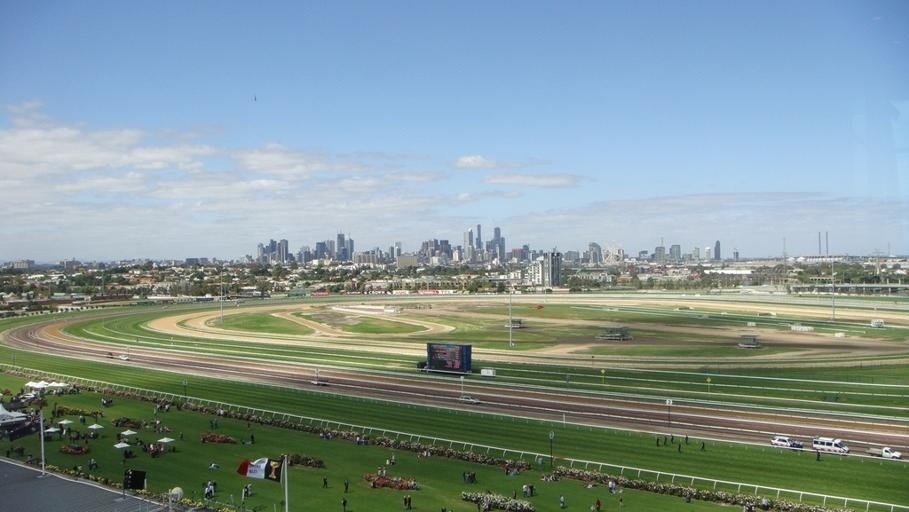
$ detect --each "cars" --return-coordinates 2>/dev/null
[458,395,480,404]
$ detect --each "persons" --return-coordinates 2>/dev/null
[1,369,858,512]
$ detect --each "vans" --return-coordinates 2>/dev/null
[812,437,850,454]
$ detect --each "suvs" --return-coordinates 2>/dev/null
[770,436,795,447]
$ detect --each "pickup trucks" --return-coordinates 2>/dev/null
[864,447,903,460]
